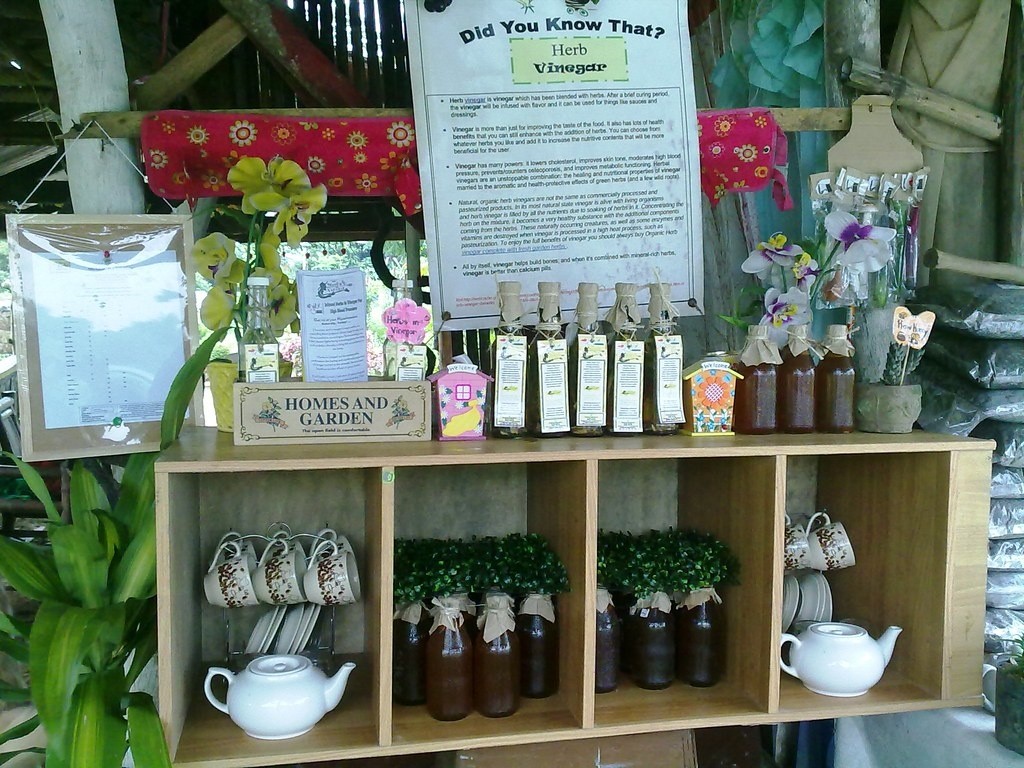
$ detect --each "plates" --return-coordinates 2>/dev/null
[245,604,322,655]
[782,572,833,634]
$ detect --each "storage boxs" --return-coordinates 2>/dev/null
[432,729,698,768]
[232,376,434,446]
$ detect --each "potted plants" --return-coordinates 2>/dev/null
[856,341,926,435]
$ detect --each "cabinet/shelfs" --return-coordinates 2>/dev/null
[154,424,997,768]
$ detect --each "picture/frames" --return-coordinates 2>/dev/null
[4,213,203,466]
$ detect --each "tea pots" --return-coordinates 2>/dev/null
[780,623,904,698]
[205,655,357,741]
[981,647,1024,716]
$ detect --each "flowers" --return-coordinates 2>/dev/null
[191,154,329,343]
[717,210,896,351]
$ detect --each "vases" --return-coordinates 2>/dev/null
[206,358,295,435]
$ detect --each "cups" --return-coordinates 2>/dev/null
[202,530,360,609]
[785,512,856,571]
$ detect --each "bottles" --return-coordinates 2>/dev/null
[394,592,557,724]
[595,587,721,695]
[491,281,683,439]
[383,280,425,381]
[733,324,856,436]
[238,277,279,383]
